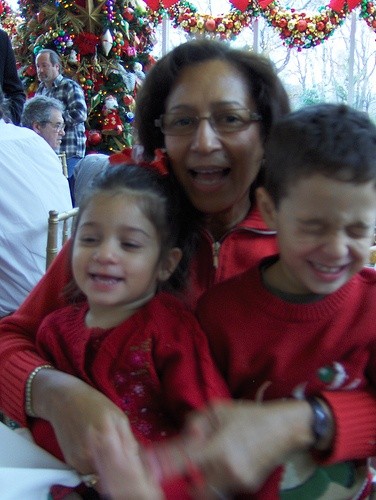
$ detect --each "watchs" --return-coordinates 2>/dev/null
[304,395,329,465]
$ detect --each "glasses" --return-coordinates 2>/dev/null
[42,122,66,132]
[155,108,261,136]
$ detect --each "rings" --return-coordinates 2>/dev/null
[83,475,101,487]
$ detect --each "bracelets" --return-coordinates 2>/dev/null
[25,364,57,418]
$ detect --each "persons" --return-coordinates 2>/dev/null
[0,38,376,500]
[0,86,73,320]
[33,49,87,178]
[19,96,67,154]
[196,103,376,500]
[0,28,27,127]
[31,160,234,500]
